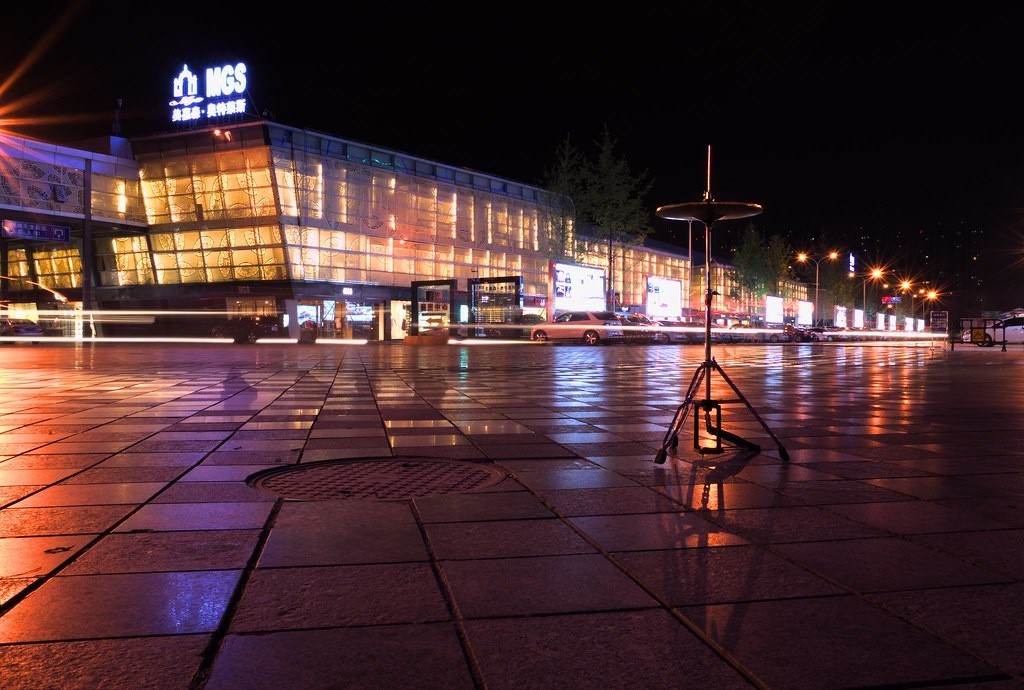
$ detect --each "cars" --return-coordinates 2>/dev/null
[805,326,947,341]
[963,317,1024,347]
[530,310,624,346]
[616,312,819,344]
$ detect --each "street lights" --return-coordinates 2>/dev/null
[847,267,884,312]
[797,249,840,326]
[885,278,940,319]
